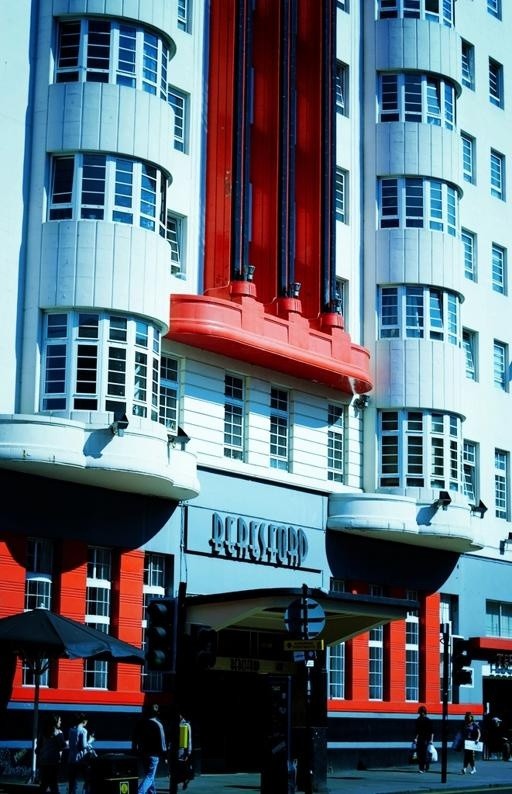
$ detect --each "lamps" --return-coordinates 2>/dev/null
[355,394,370,408]
[469,499,487,513]
[331,293,342,313]
[437,491,452,506]
[112,410,128,430]
[248,264,256,280]
[289,282,301,296]
[505,532,512,543]
[168,425,190,444]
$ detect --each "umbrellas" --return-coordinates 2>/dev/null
[0,603,146,783]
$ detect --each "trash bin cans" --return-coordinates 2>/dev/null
[91,753,140,794]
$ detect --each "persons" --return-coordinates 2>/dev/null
[488,717,511,761]
[168,709,191,790]
[132,704,167,794]
[35,714,67,794]
[70,711,94,794]
[459,712,481,774]
[413,706,436,773]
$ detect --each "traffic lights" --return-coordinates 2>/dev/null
[144,602,171,667]
[453,638,472,687]
